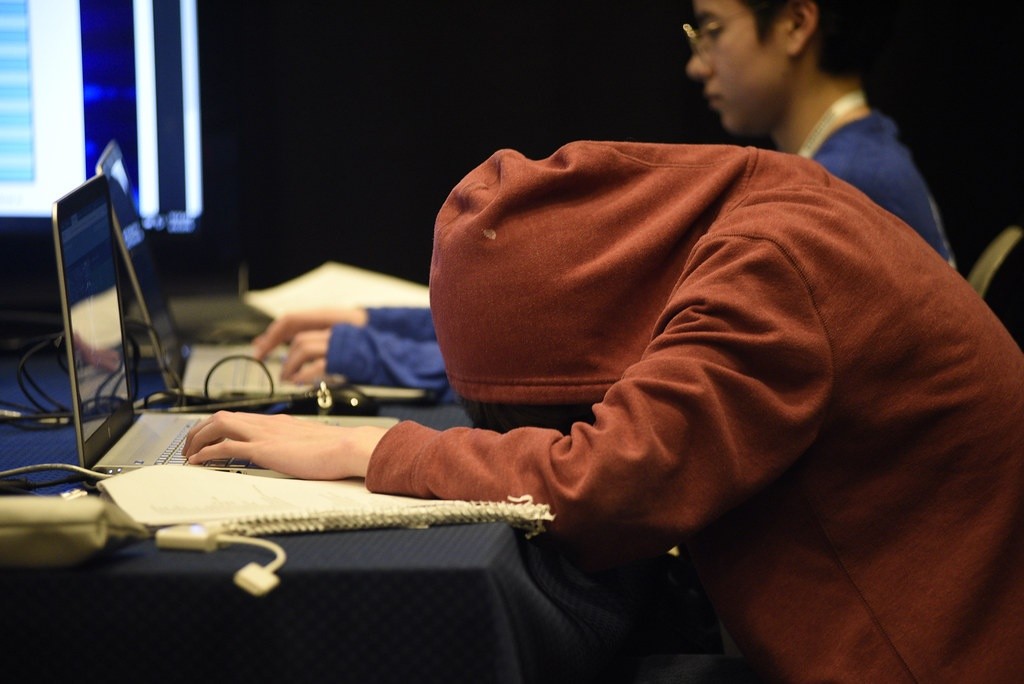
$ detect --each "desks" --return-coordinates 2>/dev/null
[0,329,594,683]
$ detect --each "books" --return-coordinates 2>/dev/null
[98,464,556,538]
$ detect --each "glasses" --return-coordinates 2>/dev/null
[683,11,752,66]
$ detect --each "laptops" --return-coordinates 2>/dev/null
[53,174,399,481]
[96,140,427,404]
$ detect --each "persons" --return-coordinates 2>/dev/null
[182,138,1024,683]
[253,0,955,400]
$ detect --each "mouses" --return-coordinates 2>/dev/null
[287,382,380,417]
